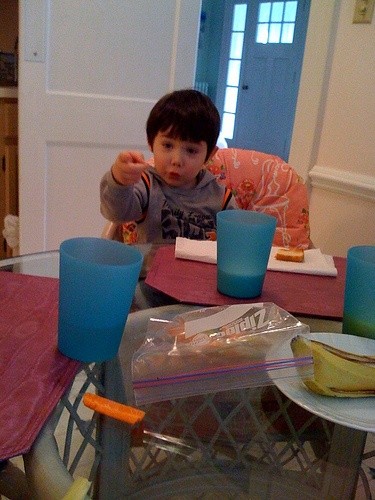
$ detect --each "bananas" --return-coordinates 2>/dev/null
[291,335,374,399]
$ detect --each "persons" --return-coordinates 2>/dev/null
[98,88,244,245]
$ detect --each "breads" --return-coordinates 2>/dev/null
[276,247,304,262]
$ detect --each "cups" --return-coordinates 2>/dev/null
[57,237,143,365]
[343,246,375,333]
[216,211,277,298]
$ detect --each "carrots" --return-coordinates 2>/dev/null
[83,393,145,426]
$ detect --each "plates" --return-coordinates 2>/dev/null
[266,332,375,433]
